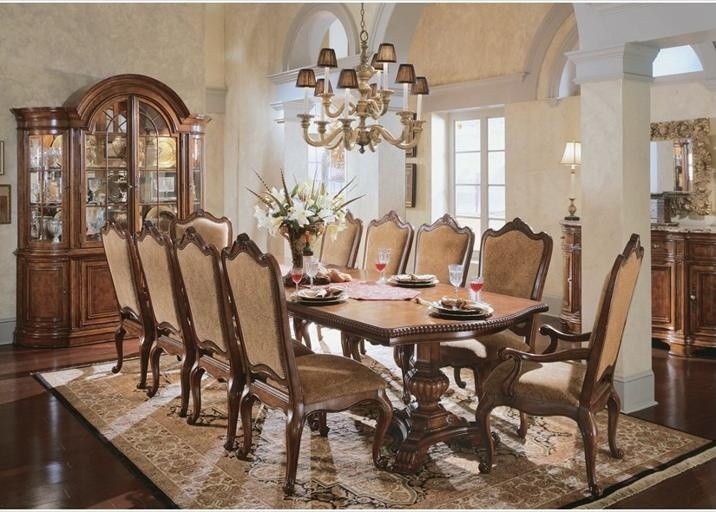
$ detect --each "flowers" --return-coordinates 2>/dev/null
[244,168,367,234]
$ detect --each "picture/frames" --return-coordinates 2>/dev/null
[0,141,4,175]
[0,184,11,225]
[403,163,417,208]
[405,112,417,158]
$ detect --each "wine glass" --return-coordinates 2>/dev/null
[374,252,388,284]
[379,249,393,283]
[470,276,484,302]
[305,258,319,288]
[88,179,99,204]
[290,268,304,291]
[448,263,464,297]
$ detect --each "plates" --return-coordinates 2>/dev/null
[291,289,348,304]
[429,300,495,319]
[388,275,438,287]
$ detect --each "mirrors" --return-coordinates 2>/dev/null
[650,135,694,196]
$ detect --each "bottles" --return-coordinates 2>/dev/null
[673,154,684,191]
[302,231,313,273]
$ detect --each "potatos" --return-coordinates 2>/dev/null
[317,266,351,282]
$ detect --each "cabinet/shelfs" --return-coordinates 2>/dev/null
[8,73,211,351]
[558,224,581,336]
[652,220,716,360]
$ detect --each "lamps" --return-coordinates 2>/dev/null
[294,3,430,155]
[560,140,581,222]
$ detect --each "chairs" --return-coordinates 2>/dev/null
[221,232,393,497]
[173,226,270,451]
[171,211,234,252]
[476,233,645,499]
[462,218,555,438]
[318,201,474,399]
[132,221,215,419]
[99,215,156,390]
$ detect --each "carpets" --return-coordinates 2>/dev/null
[31,347,716,509]
